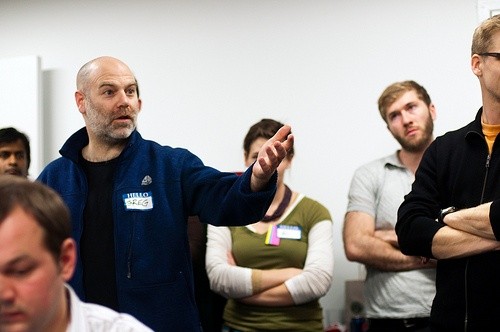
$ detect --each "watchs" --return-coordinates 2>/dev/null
[435,206,457,220]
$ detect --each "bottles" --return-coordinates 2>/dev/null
[350,315,364,332]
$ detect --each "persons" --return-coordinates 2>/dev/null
[31,56,295,332]
[342,80,441,332]
[0,127,30,178]
[1,175,156,332]
[393,15,500,332]
[206,118,333,332]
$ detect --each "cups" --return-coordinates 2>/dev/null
[326,309,344,332]
